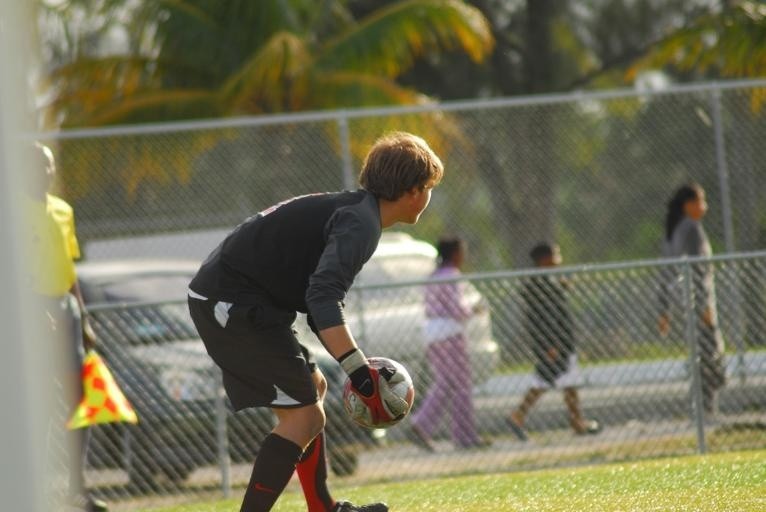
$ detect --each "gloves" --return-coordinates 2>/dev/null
[338,349,409,427]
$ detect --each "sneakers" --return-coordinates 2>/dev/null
[455,437,495,451]
[66,490,108,511]
[409,422,438,454]
[504,417,529,442]
[334,501,389,512]
[576,419,602,435]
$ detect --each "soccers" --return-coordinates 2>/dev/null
[344,357,413,430]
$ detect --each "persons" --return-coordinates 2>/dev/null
[0,139,109,512]
[186,132,445,512]
[656,184,729,425]
[504,240,605,441]
[407,236,492,453]
